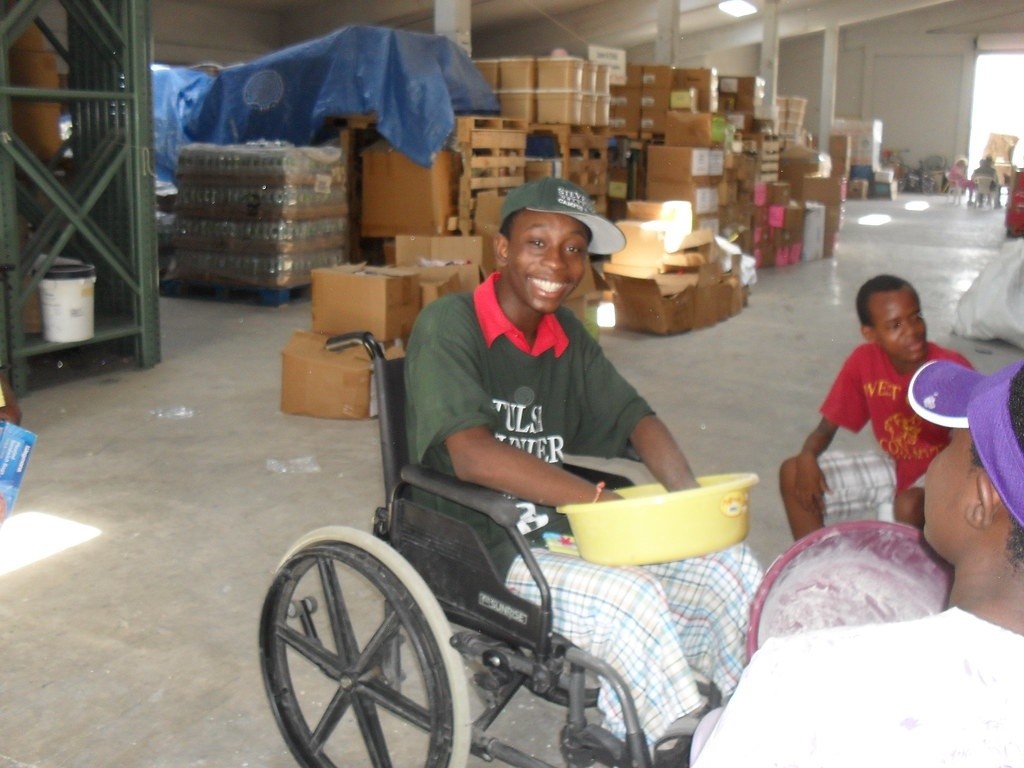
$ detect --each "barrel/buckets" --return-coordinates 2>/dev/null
[31,263,97,343]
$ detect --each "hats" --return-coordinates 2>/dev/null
[907,359,1024,529]
[499,177,626,255]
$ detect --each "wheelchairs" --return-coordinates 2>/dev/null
[248,327,719,768]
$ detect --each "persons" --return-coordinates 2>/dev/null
[685,358,1024,766]
[780,272,981,539]
[948,155,1004,209]
[400,178,760,764]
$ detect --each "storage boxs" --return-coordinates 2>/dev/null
[165,22,902,422]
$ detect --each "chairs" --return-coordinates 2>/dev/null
[972,173,1002,209]
[944,172,965,206]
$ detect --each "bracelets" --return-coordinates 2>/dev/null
[594,479,606,503]
